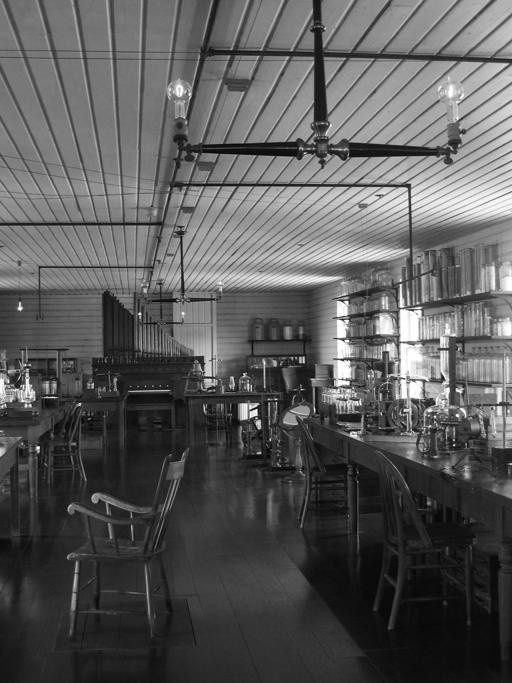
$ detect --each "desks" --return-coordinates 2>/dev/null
[62,388,130,461]
[1,399,82,537]
[184,391,285,433]
[303,414,512,683]
[0,436,23,549]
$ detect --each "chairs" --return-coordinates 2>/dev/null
[293,416,356,530]
[66,448,192,642]
[40,404,88,485]
[373,449,477,632]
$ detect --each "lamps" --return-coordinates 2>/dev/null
[137,310,186,328]
[16,297,24,312]
[140,280,224,303]
[163,76,465,165]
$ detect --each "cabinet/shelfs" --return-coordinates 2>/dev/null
[403,290,511,410]
[331,285,401,384]
[247,336,312,359]
